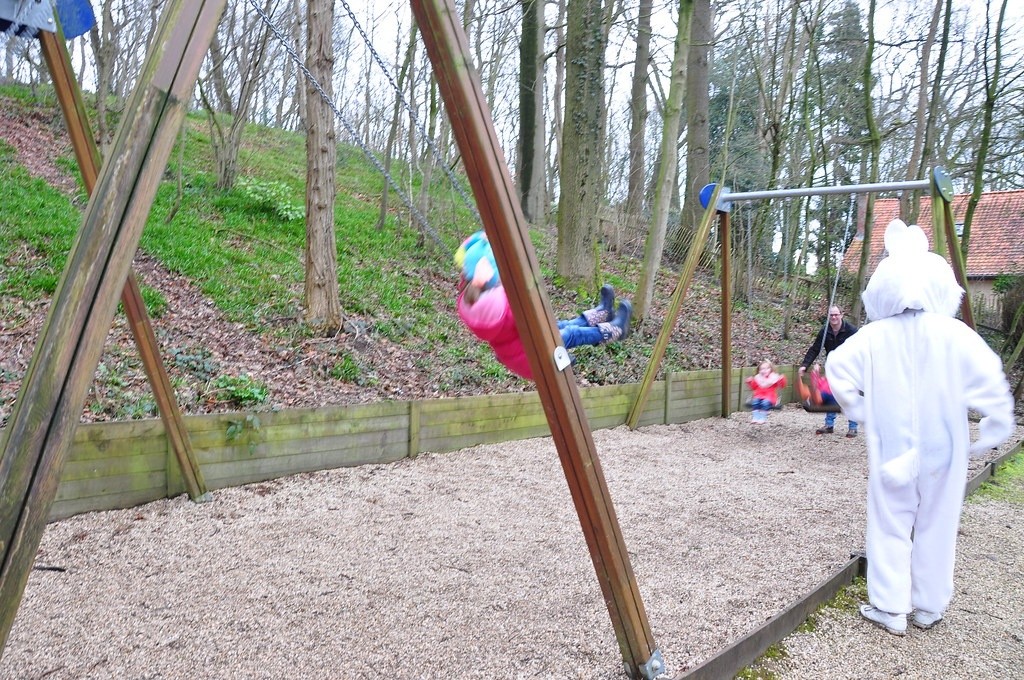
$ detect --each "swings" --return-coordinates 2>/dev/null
[744,203,792,412]
[801,198,900,415]
[250,1,577,366]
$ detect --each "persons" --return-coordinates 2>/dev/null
[798,364,837,408]
[744,359,786,424]
[450,228,633,385]
[798,305,859,437]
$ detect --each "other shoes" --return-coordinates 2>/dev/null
[846,429,858,438]
[816,425,833,434]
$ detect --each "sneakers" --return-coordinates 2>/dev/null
[913,609,942,629]
[861,605,907,635]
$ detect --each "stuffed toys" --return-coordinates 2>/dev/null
[825,218,1014,637]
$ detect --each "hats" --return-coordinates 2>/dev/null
[454,229,503,288]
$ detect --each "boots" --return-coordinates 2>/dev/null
[750,410,759,423]
[596,299,632,344]
[582,284,616,327]
[756,410,767,424]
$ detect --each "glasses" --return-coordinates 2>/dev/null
[830,313,841,318]
